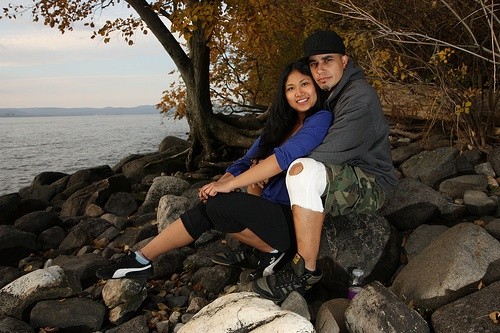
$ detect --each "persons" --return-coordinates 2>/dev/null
[212,30,398,301]
[96,61,333,281]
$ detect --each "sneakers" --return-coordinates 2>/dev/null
[212,249,254,267]
[95,250,155,279]
[259,251,286,277]
[254,251,323,303]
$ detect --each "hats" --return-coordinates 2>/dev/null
[297,29,347,61]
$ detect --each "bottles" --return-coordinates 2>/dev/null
[349,268,367,300]
